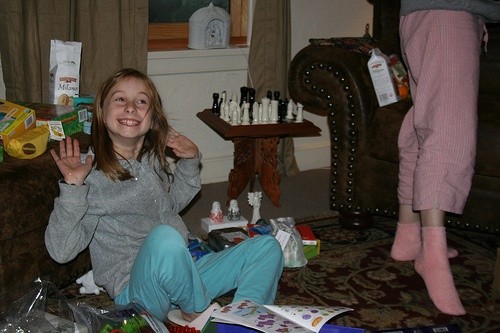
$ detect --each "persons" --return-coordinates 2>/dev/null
[390,0,500,316]
[44,68,284,322]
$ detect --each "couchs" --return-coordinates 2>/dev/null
[287,0,500,227]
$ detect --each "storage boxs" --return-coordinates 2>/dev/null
[0,97,96,151]
[200,214,321,260]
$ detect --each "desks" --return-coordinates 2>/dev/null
[0,116,95,321]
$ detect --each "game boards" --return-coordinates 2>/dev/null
[196,108,321,138]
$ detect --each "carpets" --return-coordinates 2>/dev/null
[43,209,500,333]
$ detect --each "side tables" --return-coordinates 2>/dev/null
[195,109,322,207]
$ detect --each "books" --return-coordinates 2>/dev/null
[209,300,353,333]
